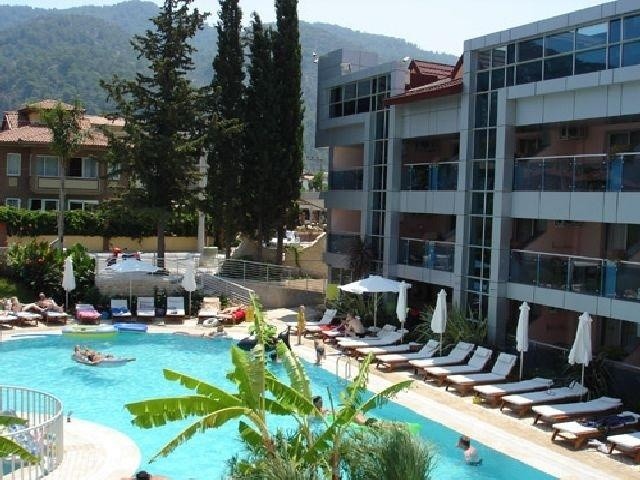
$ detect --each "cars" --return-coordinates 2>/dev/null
[268,230,301,249]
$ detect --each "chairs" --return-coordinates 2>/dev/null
[288,308,422,361]
[606,430,640,461]
[549,411,640,449]
[532,396,624,426]
[425,345,494,388]
[75,303,102,325]
[198,297,219,323]
[409,341,475,380]
[500,381,588,416]
[111,299,131,325]
[166,296,186,327]
[137,297,155,325]
[0,299,67,330]
[471,377,555,409]
[377,339,441,373]
[445,352,518,397]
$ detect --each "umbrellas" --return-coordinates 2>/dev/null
[430,289,447,357]
[395,280,408,343]
[107,255,164,308]
[337,274,411,329]
[183,255,197,313]
[61,255,76,311]
[515,301,530,380]
[568,312,593,385]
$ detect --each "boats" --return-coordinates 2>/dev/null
[76,354,136,366]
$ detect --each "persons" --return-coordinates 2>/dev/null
[75,345,103,356]
[11,296,41,313]
[345,314,365,338]
[89,354,113,361]
[38,293,63,313]
[310,395,332,420]
[297,305,307,345]
[455,434,479,464]
